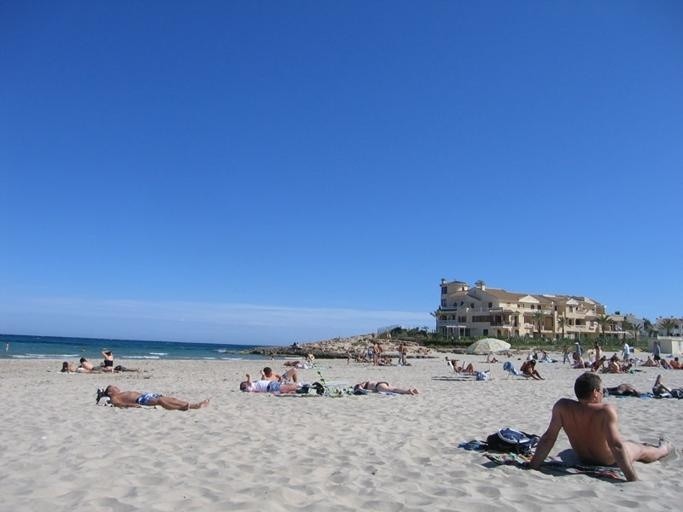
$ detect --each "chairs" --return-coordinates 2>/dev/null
[444,356,480,380]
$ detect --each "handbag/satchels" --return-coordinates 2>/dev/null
[485,425,541,455]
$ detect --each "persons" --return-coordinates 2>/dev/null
[78,358,116,373]
[62,362,101,373]
[102,350,113,367]
[528,374,668,482]
[96,385,210,411]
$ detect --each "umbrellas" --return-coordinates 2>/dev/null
[467,337,512,354]
